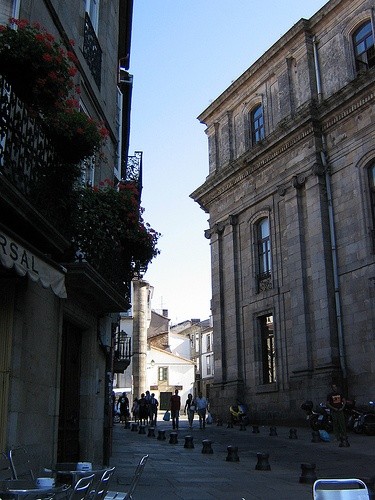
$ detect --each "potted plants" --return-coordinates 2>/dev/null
[0,15,163,306]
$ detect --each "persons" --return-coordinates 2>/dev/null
[228,402,247,423]
[133,390,158,425]
[194,391,210,428]
[165,389,181,428]
[326,383,352,448]
[183,393,198,427]
[116,392,130,423]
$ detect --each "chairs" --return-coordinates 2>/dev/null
[91,467,116,500]
[9,446,35,480]
[313,478,369,500]
[105,454,149,500]
[0,451,14,480]
[69,474,96,500]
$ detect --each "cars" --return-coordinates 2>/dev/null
[113,402,129,424]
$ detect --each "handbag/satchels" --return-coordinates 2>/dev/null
[206,413,212,424]
[319,429,332,442]
[163,410,170,421]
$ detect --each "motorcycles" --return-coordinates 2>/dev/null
[301,398,375,436]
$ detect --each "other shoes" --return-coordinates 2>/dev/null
[337,439,344,447]
[346,439,352,447]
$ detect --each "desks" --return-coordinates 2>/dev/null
[0,477,72,500]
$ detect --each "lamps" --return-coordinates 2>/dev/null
[119,329,128,344]
